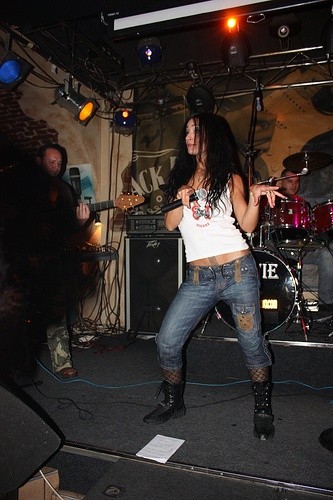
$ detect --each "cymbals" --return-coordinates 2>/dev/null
[282,150,333,175]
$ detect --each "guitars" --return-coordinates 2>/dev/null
[0,193,148,246]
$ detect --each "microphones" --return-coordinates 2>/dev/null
[161,189,208,213]
[255,78,262,111]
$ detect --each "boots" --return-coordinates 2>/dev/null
[143,380,186,425]
[252,381,275,440]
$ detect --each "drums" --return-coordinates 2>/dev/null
[311,199,333,240]
[211,247,300,337]
[264,243,271,243]
[266,200,309,243]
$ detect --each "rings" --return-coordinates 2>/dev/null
[266,191,271,193]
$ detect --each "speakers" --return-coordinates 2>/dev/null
[124,235,186,336]
[0,385,62,493]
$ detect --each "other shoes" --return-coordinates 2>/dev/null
[62,367,78,377]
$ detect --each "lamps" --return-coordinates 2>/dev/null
[0,14,250,137]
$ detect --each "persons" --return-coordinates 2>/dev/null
[259,168,333,312]
[36,144,91,380]
[142,111,286,440]
